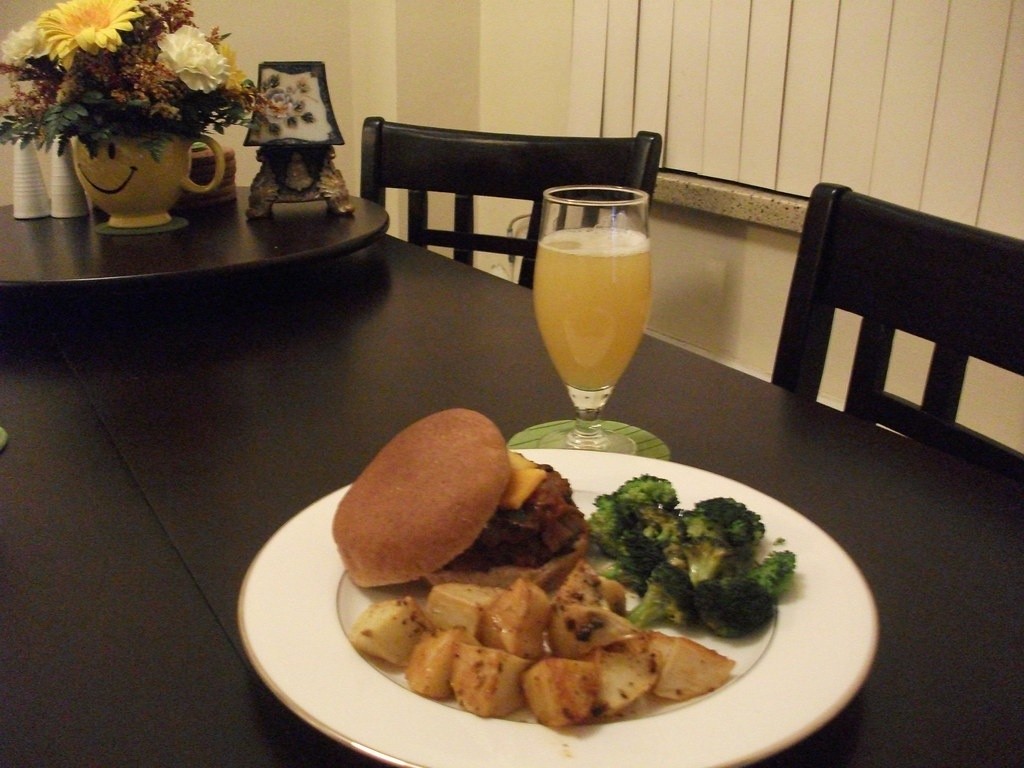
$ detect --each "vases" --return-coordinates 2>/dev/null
[68,127,227,228]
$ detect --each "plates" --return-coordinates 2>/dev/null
[238,449,879,768]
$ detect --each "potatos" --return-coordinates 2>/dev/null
[347,564,738,728]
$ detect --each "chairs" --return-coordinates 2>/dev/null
[770,182,1024,483]
[360,116,663,289]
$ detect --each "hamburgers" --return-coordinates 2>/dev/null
[331,408,588,587]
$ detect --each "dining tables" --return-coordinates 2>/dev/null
[0,231,1024,768]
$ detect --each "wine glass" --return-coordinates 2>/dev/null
[533,185,651,455]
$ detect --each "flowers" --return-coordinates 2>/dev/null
[0,0,290,161]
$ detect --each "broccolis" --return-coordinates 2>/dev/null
[590,474,796,635]
